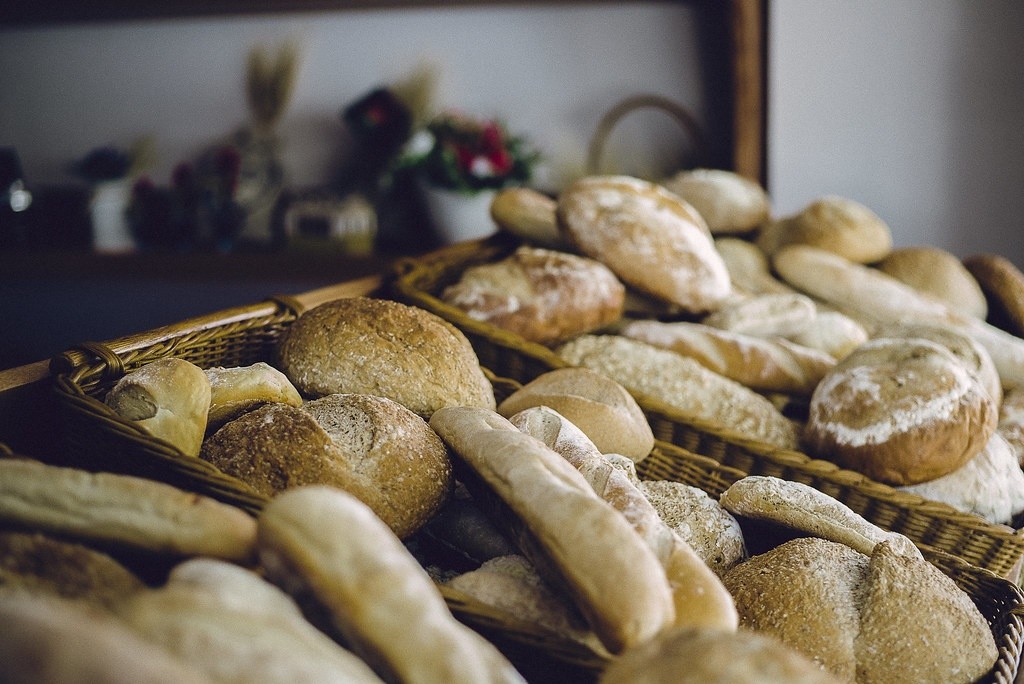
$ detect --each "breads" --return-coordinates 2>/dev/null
[0,167,1024,684]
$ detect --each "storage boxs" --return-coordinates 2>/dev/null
[1,233,1024,684]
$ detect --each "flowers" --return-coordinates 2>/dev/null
[394,110,543,195]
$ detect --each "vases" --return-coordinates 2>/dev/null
[418,172,503,249]
